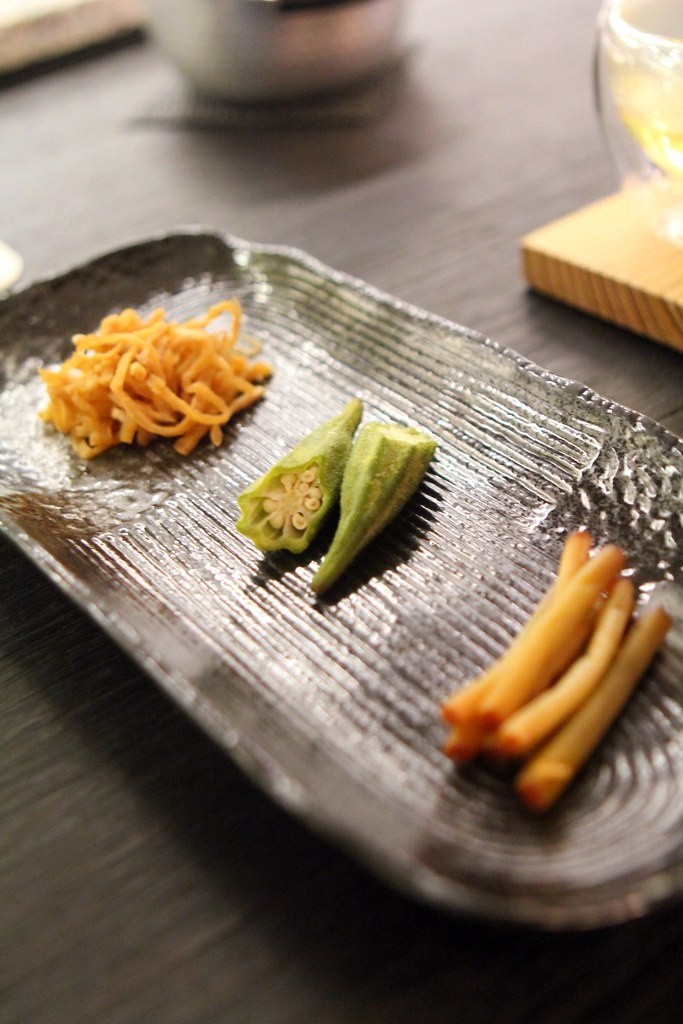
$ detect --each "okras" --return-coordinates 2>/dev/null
[310,424,437,590]
[237,400,364,550]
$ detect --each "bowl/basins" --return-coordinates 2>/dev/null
[127,0,406,104]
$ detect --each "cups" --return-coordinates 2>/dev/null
[594,0,683,247]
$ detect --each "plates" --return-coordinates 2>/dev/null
[0,227,683,928]
